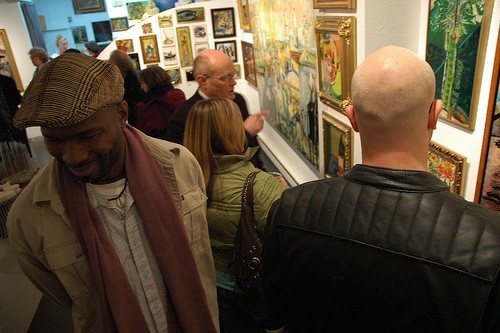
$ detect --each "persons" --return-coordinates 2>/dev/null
[6,52,220,333]
[28,35,186,145]
[266,45,500,333]
[0,75,40,178]
[173,50,267,172]
[183,98,291,333]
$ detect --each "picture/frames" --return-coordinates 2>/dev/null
[72,0,105,15]
[194,42,209,57]
[314,15,357,115]
[0,28,24,93]
[140,35,160,64]
[322,111,354,179]
[158,15,173,28]
[427,140,467,196]
[241,41,257,86]
[215,40,238,63]
[313,0,356,9]
[474,21,500,210]
[237,0,251,30]
[175,26,194,67]
[425,0,495,131]
[110,17,129,32]
[232,65,241,79]
[211,8,236,38]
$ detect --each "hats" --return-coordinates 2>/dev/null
[13,52,126,131]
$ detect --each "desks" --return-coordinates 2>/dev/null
[0,168,40,238]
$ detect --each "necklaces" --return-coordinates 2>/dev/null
[108,173,129,202]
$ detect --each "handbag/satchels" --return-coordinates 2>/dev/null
[234,171,265,296]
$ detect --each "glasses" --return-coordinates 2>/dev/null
[206,72,236,83]
